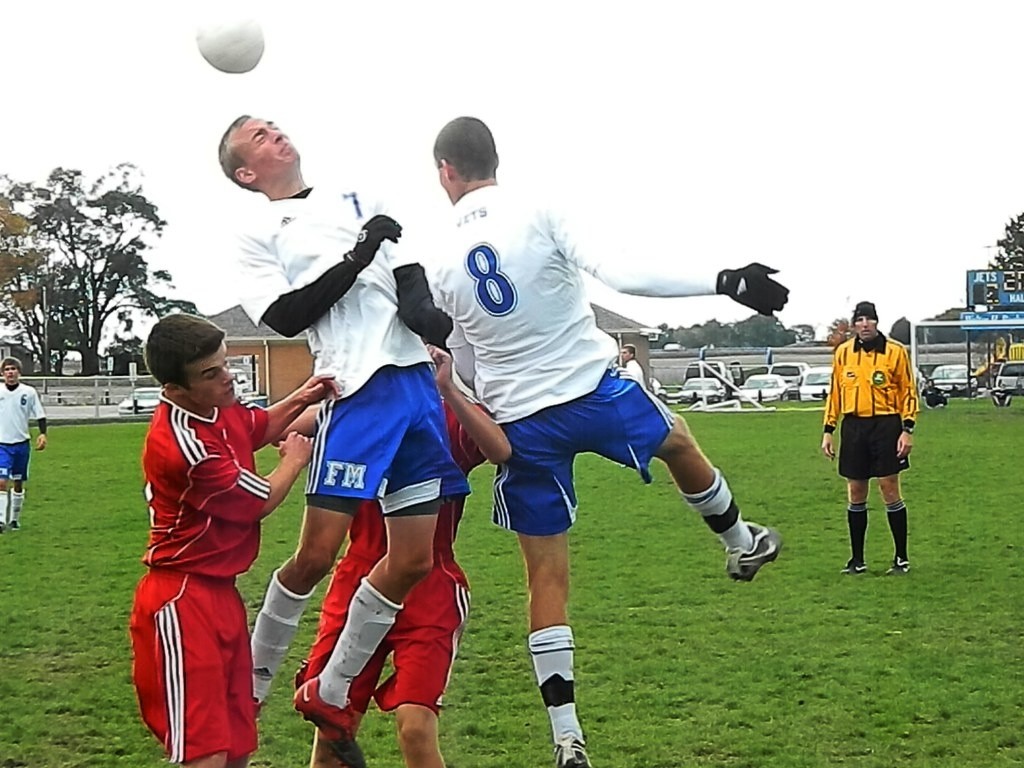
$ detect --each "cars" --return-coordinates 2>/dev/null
[798,366,836,402]
[928,364,979,400]
[647,376,668,404]
[737,373,790,404]
[994,358,1024,396]
[683,359,764,394]
[118,387,162,414]
[675,376,728,407]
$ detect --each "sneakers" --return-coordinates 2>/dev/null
[252,697,264,719]
[294,676,366,768]
[842,558,868,575]
[0,522,4,531]
[885,556,910,576]
[8,520,20,531]
[726,521,782,583]
[557,733,592,768]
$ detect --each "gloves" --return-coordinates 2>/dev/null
[344,215,402,274]
[715,262,790,315]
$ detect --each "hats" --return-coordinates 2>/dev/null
[852,302,879,327]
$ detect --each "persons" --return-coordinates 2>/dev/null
[622,344,643,386]
[0,357,47,534]
[272,343,511,768]
[425,117,790,768]
[218,114,453,768]
[820,302,921,576]
[130,313,342,768]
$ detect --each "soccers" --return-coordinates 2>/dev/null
[196,26,265,74]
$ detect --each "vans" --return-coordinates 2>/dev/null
[768,360,815,399]
